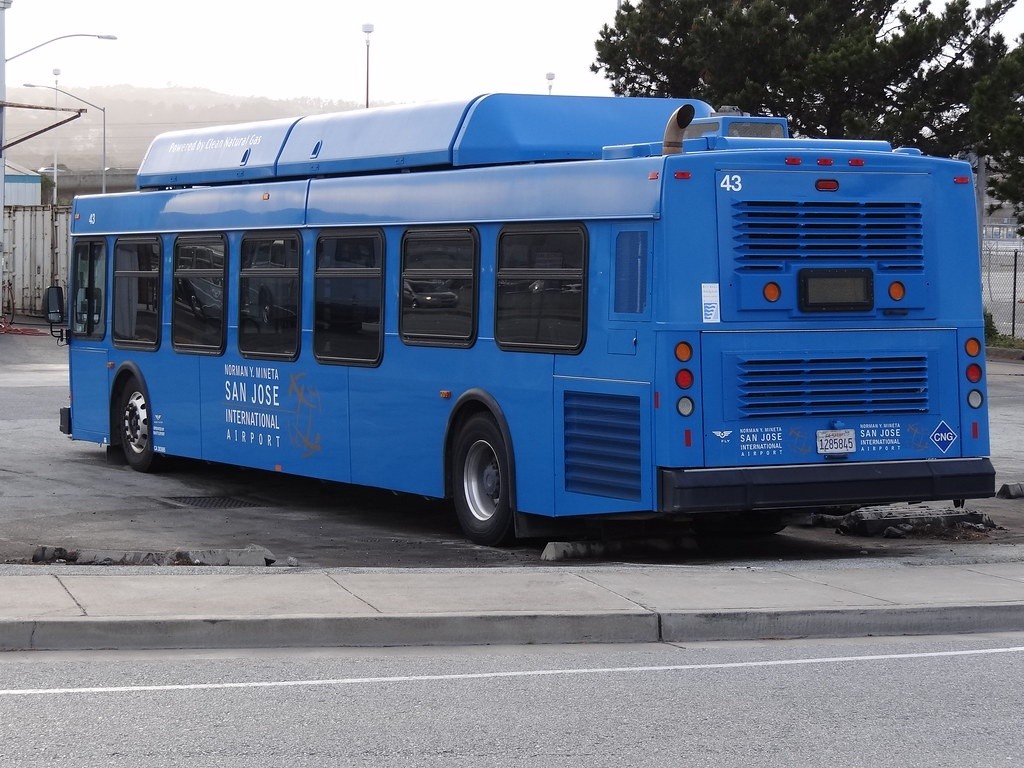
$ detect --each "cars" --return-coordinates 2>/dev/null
[497,277,583,308]
[399,276,459,310]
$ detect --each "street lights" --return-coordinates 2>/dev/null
[22,81,107,193]
[0,32,118,296]
[546,72,559,96]
[51,68,61,205]
[363,22,376,110]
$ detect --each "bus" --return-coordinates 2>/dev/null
[40,91,999,556]
